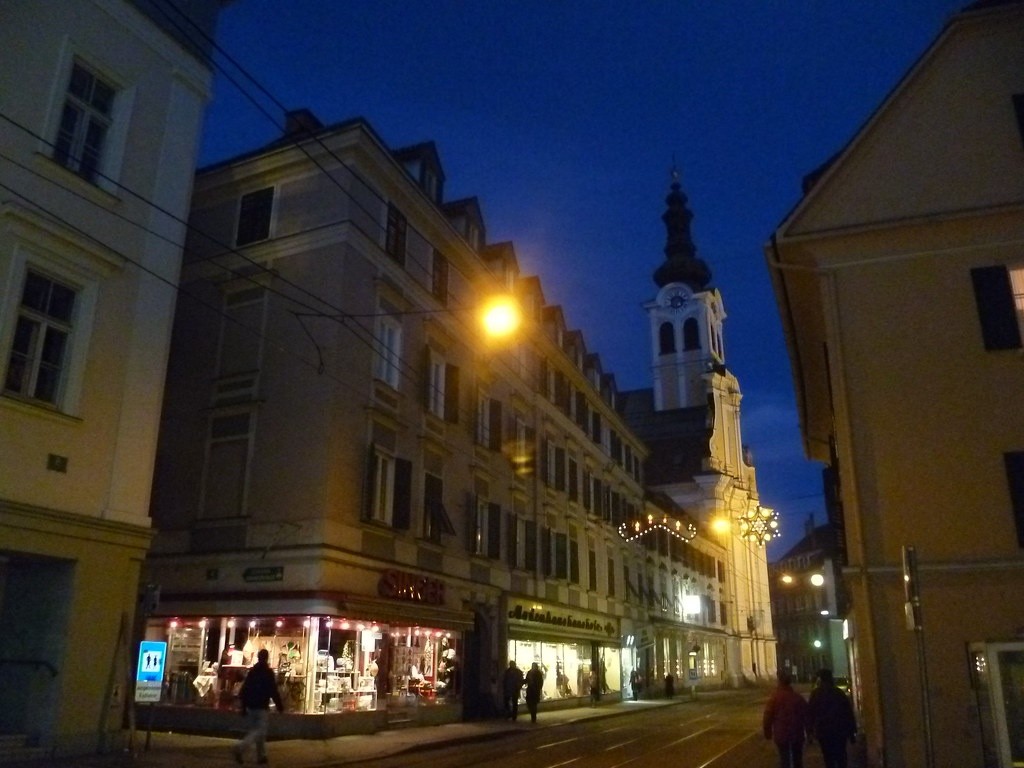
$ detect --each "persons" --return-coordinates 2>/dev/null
[523,662,543,721]
[630,670,639,701]
[665,673,674,700]
[588,671,601,707]
[502,660,523,721]
[807,667,858,768]
[763,666,812,768]
[231,648,286,766]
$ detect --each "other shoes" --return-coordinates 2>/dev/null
[258,757,267,763]
[233,748,244,765]
